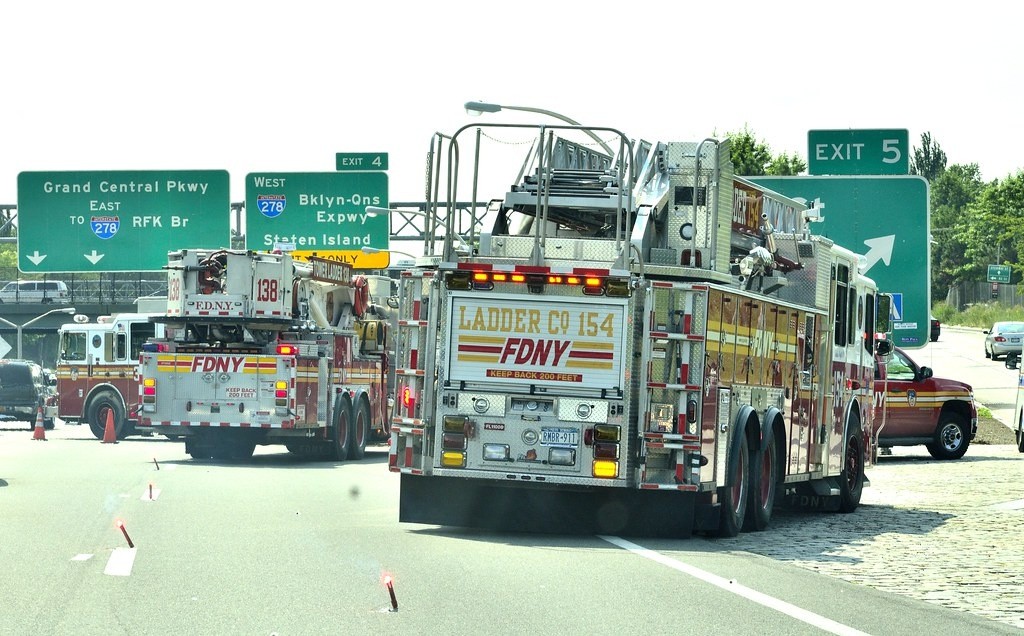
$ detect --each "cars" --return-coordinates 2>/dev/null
[929,315,941,343]
[983,321,1024,361]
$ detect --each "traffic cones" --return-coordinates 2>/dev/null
[30,406,48,441]
[100,408,119,444]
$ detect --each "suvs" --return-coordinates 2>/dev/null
[865,342,979,460]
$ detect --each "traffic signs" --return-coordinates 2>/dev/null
[986,264,1011,284]
[16,169,230,275]
[242,152,390,253]
[732,127,933,350]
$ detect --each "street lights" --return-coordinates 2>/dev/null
[0,307,76,359]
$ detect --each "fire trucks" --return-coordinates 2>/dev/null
[131,247,416,462]
[385,122,894,541]
[54,312,170,441]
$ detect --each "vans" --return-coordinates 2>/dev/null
[0,358,59,431]
[0,279,69,306]
